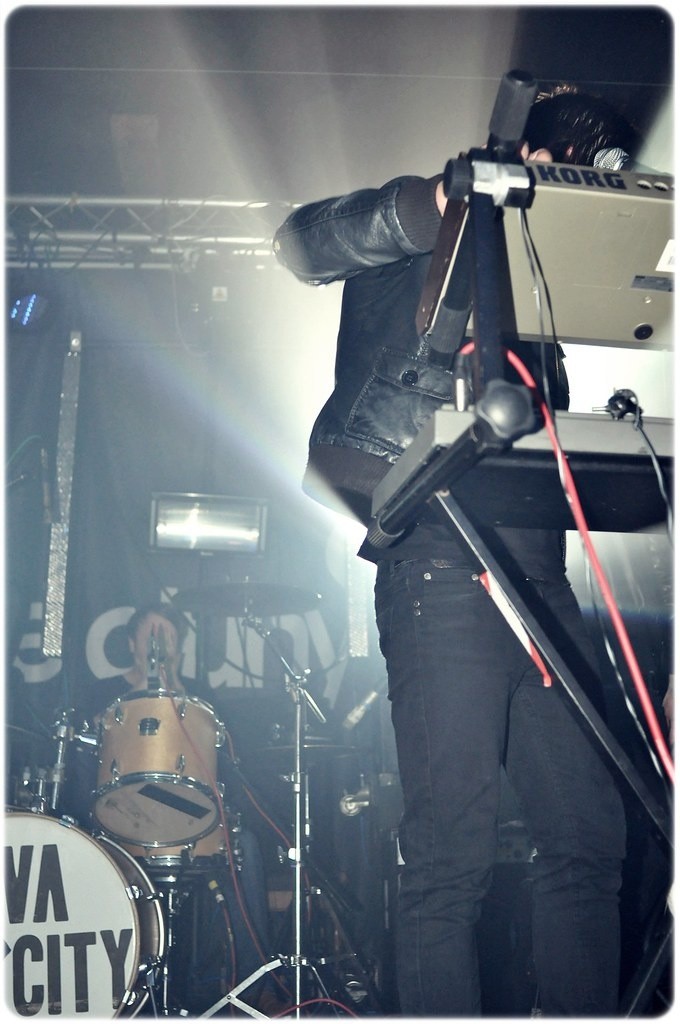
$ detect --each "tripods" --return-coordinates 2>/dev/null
[195,595,387,1020]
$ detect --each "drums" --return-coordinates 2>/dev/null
[89,688,223,849]
[119,821,234,885]
[5,812,166,1020]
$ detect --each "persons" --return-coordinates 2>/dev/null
[273,86,629,1018]
[68,604,222,839]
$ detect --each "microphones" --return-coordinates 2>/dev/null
[592,147,662,173]
[146,624,160,690]
[40,444,53,524]
[342,675,389,730]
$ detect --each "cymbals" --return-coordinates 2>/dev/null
[171,580,322,618]
[250,742,364,761]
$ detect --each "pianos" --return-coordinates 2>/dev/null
[370,398,674,536]
[414,147,676,354]
